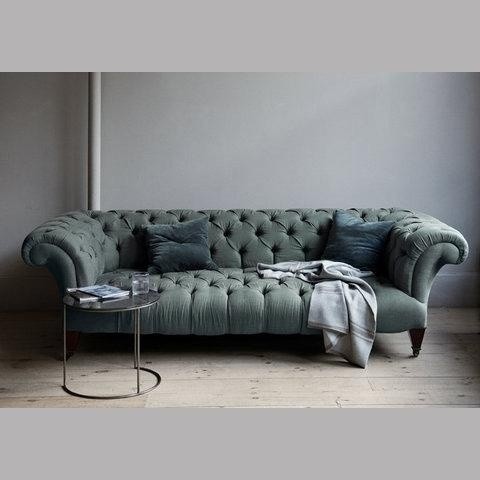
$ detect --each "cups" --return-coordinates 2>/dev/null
[132,271,149,295]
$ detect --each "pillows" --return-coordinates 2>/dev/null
[322,210,396,271]
[141,214,219,276]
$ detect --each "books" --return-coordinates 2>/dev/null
[98,297,130,303]
[66,284,130,304]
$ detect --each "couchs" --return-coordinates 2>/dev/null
[20,207,470,363]
